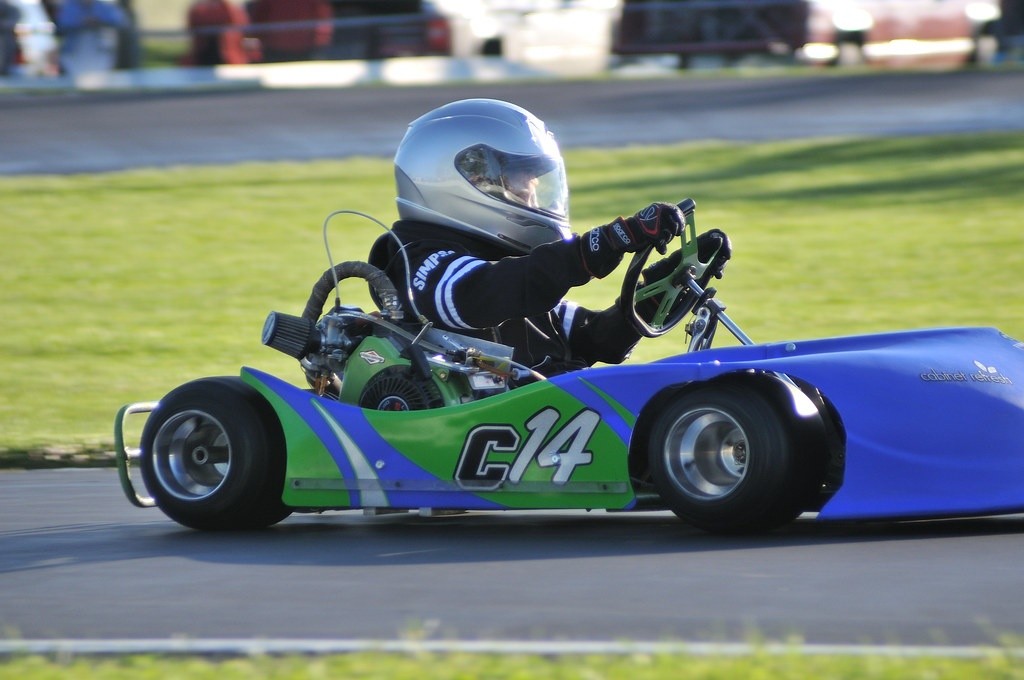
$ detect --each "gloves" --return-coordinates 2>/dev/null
[581,202,686,280]
[642,228,733,307]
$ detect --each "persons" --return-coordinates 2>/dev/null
[256,1,332,63]
[54,0,128,72]
[186,0,248,64]
[366,97,731,390]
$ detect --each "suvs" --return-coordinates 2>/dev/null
[0,0,141,77]
[609,0,1005,70]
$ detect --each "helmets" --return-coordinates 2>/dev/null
[392,97,573,254]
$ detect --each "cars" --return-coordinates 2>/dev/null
[188,0,452,61]
